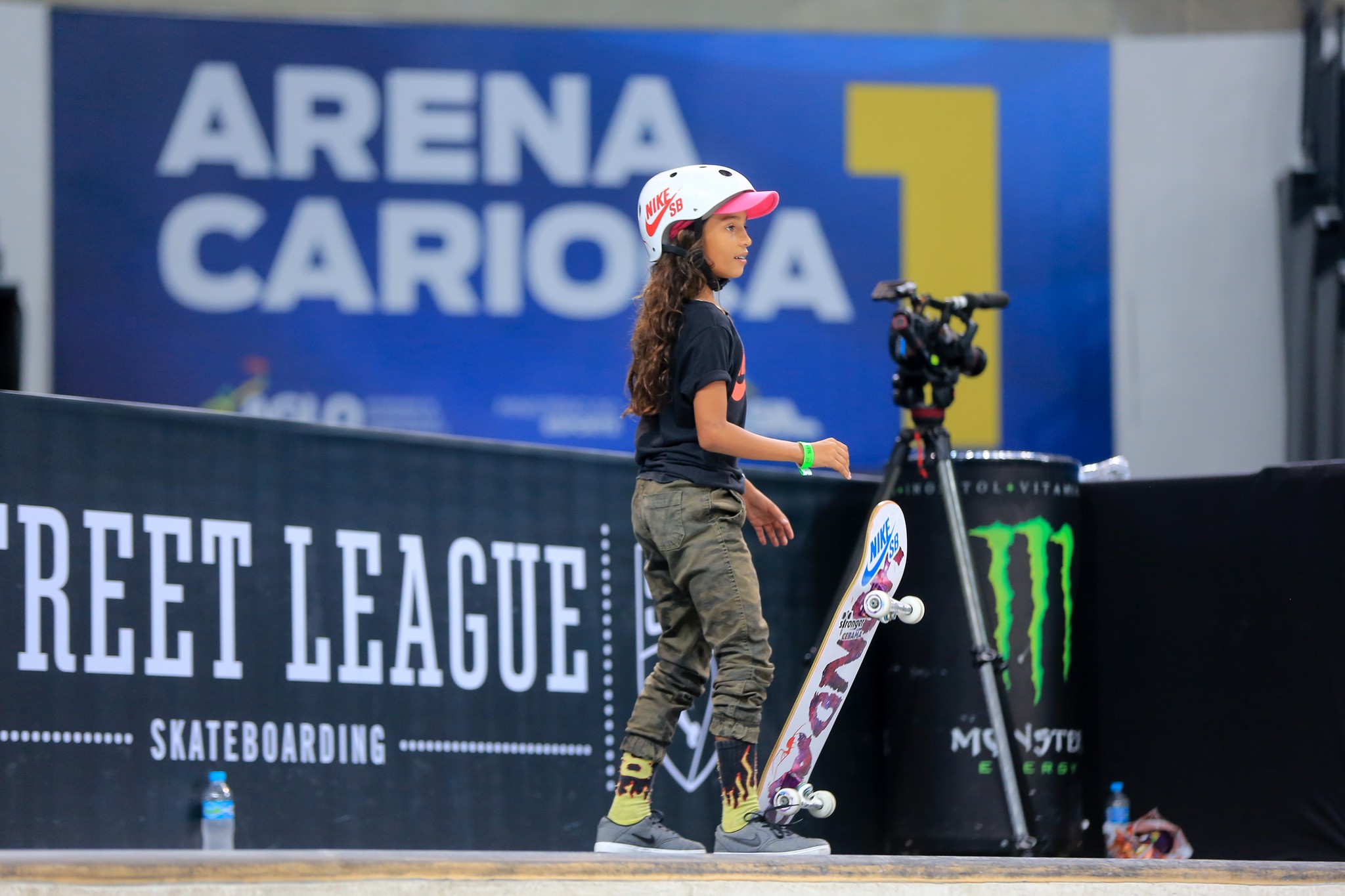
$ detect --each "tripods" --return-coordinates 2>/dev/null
[802,400,1037,854]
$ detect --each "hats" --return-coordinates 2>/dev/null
[671,189,779,238]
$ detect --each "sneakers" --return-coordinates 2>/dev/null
[713,804,831,857]
[594,809,706,856]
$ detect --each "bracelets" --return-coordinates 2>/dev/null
[795,440,814,476]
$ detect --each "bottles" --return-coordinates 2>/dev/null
[1106,782,1129,857]
[1079,455,1131,482]
[201,771,235,850]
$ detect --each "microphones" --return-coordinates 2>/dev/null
[962,291,1009,310]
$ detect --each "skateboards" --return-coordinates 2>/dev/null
[757,499,924,831]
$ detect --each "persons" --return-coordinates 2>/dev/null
[590,164,851,855]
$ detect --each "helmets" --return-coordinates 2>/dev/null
[638,165,756,262]
